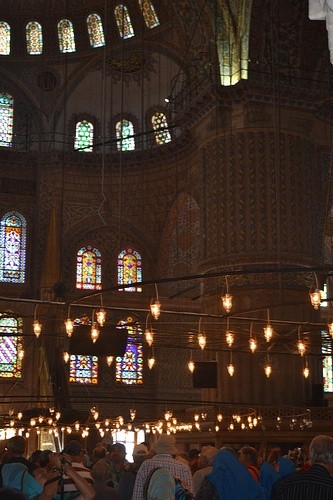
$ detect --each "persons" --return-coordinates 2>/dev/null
[0,432,310,500]
[272,435,333,500]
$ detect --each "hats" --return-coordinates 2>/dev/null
[132,444,149,456]
[153,434,178,454]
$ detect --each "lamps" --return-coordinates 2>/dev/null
[19,271,321,378]
